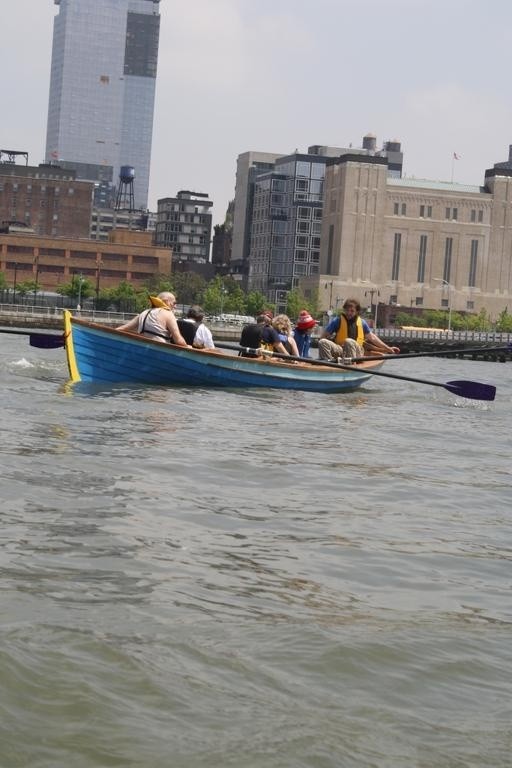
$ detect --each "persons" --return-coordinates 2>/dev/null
[292,310,316,358]
[318,298,399,365]
[240,311,299,364]
[114,291,187,346]
[170,304,214,349]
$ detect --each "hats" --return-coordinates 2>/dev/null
[297,311,315,330]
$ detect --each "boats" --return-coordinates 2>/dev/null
[62,308,386,394]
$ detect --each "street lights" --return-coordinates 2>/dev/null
[361,280,378,329]
[431,278,453,332]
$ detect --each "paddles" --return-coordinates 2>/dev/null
[30,333,67,349]
[215,343,497,400]
[308,343,512,365]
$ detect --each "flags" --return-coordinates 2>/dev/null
[453,152,461,160]
